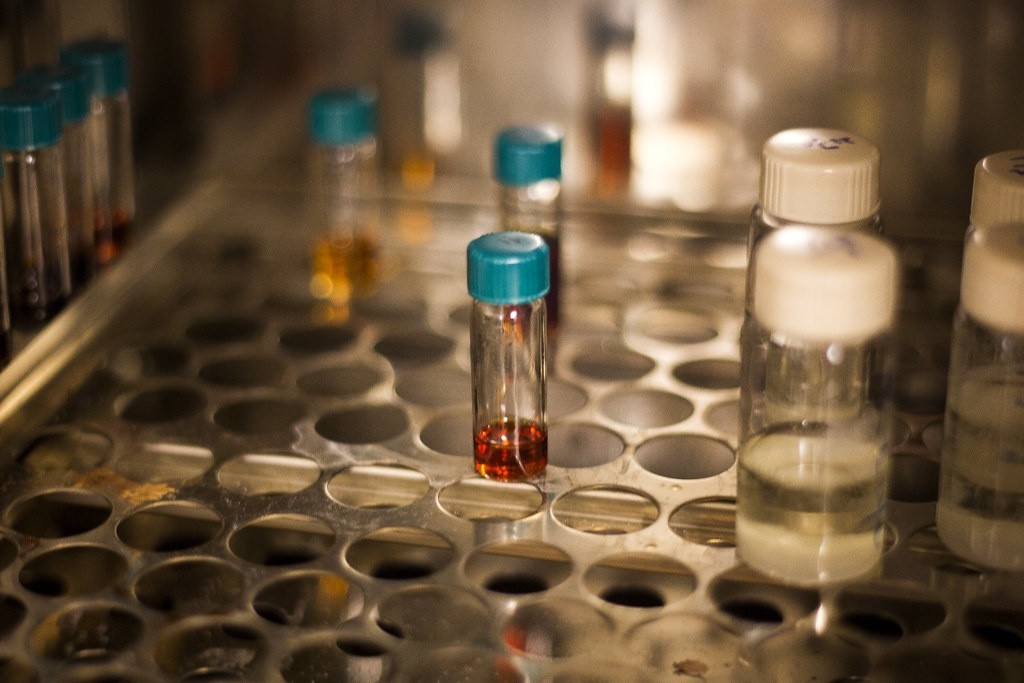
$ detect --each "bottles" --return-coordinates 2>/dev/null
[0,38,137,331]
[298,84,395,338]
[493,125,569,334]
[936,148,1024,574]
[735,127,898,588]
[466,231,551,484]
